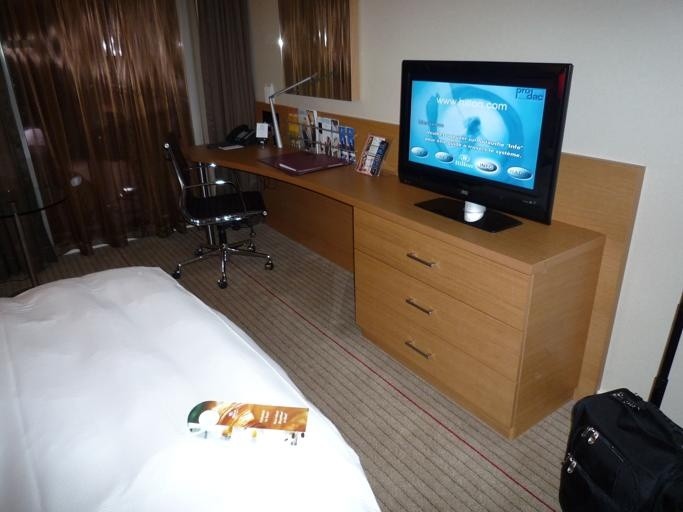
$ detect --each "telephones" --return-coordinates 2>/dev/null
[225,124,258,146]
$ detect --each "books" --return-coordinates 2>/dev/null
[272,150,346,176]
[297,106,389,177]
[177,401,310,449]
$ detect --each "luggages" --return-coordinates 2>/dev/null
[559,385,683,512]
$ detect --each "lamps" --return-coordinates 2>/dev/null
[268,71,320,151]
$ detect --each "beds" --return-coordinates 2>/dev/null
[1,264,383,512]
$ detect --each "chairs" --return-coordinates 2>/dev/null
[160,129,273,290]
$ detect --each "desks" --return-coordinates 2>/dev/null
[188,141,605,443]
[1,168,74,295]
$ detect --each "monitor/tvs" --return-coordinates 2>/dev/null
[399,60,574,233]
